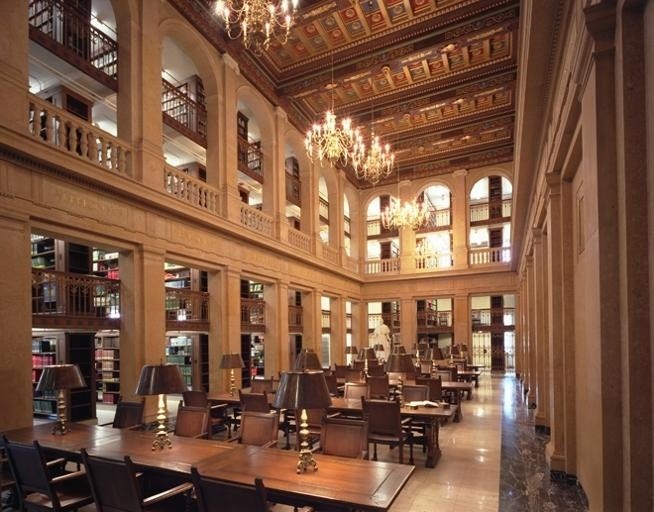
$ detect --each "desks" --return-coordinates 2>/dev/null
[203,442,415,508]
[471,361,486,382]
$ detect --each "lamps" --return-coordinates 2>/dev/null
[135,356,189,452]
[304,54,439,233]
[292,342,470,409]
[35,360,87,435]
[215,0,298,57]
[219,351,246,398]
[272,364,333,476]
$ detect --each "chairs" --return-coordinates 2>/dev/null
[0,436,274,511]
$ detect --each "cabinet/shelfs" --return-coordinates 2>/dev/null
[31,236,264,324]
[162,84,189,128]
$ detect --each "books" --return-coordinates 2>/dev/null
[31,243,59,414]
[164,262,193,386]
[91,250,120,401]
[249,279,264,381]
